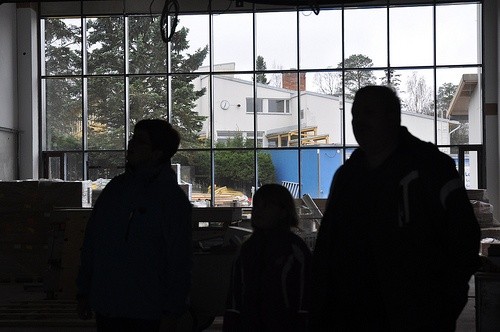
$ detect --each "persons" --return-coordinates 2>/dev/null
[75,119,193,332]
[222,86,481,332]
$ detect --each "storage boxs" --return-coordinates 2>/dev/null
[467,189,500,259]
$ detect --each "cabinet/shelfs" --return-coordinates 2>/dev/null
[189,206,253,330]
[0,179,94,298]
